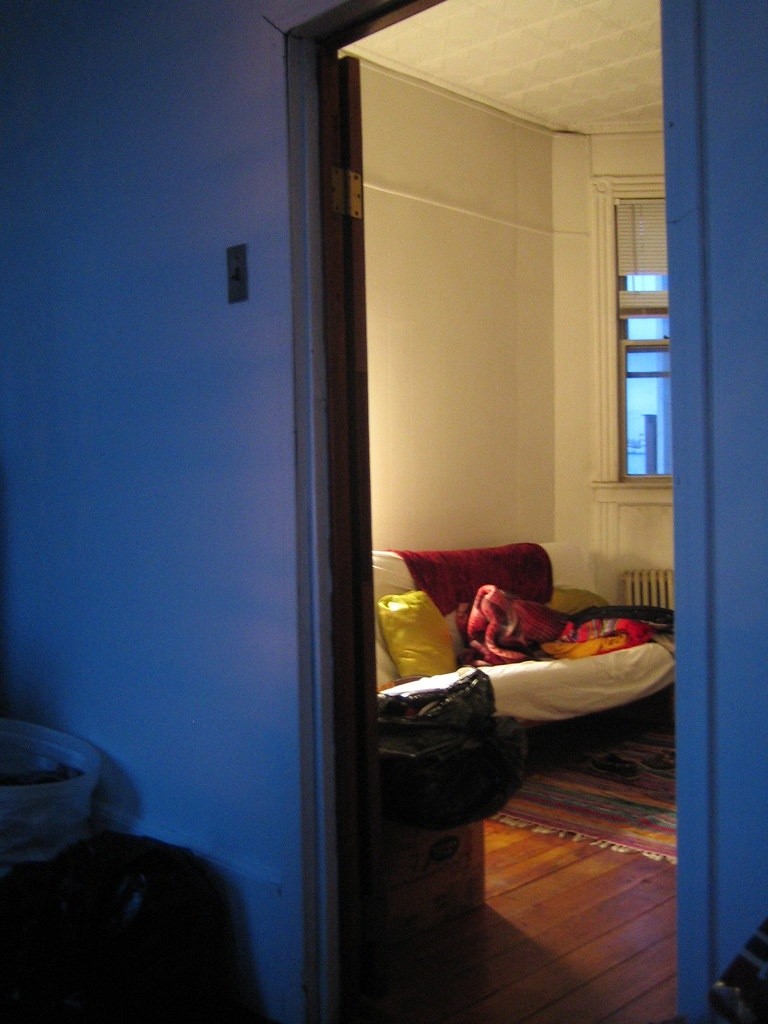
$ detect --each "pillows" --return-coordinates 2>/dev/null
[378,589,457,677]
[548,587,608,614]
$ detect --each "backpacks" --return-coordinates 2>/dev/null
[0,832,275,1023]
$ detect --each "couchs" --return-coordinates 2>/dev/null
[371,543,675,763]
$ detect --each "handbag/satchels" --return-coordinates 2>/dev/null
[378,673,498,734]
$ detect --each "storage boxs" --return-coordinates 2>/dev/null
[379,820,486,945]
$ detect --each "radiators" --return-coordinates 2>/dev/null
[619,568,674,610]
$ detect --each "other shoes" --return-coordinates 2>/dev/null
[642,749,675,768]
[580,749,638,785]
